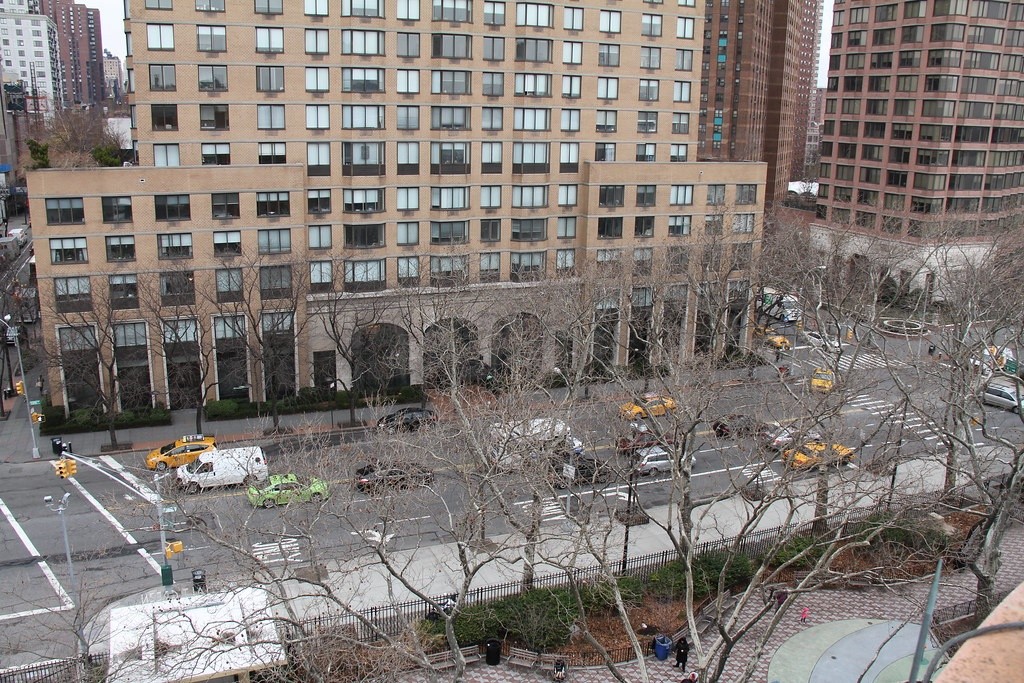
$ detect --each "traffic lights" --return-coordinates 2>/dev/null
[32,413,46,425]
[56,463,67,480]
[16,381,23,395]
[68,460,77,475]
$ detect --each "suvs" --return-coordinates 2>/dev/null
[145,437,218,471]
[542,458,612,489]
[974,379,1024,414]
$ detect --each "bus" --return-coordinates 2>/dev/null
[754,286,802,321]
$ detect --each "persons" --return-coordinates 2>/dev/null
[774,346,780,363]
[673,637,689,672]
[774,588,788,615]
[801,607,809,624]
[36,374,45,395]
[681,672,698,683]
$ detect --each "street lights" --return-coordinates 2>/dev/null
[621,422,647,577]
[796,265,826,320]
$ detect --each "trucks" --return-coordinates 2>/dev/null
[483,418,585,473]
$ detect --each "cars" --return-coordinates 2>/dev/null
[810,366,836,394]
[246,475,334,508]
[753,324,791,350]
[767,427,855,473]
[4,325,28,347]
[377,407,439,435]
[619,395,678,421]
[950,345,1014,376]
[712,413,777,441]
[352,461,435,494]
[616,429,696,477]
[801,330,842,352]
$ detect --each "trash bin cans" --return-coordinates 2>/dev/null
[653,636,672,659]
[50,436,62,453]
[192,569,207,592]
[484,638,502,666]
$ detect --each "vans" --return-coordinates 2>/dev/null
[0,229,27,260]
[170,445,268,495]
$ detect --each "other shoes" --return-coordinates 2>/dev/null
[682,668,685,672]
[673,665,679,667]
